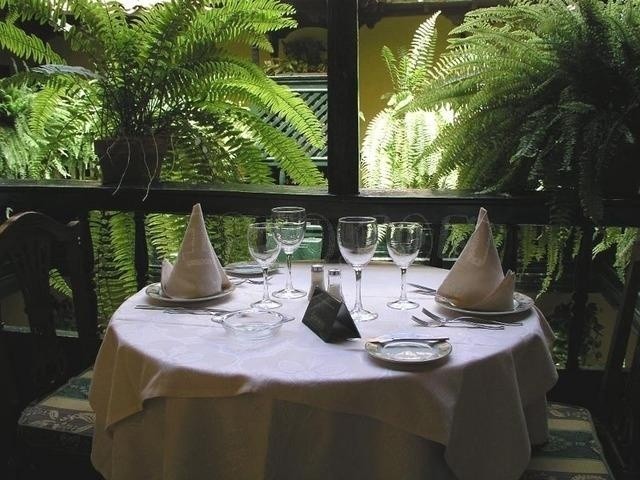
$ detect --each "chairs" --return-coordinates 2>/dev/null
[521,259,640,480]
[1,209,105,479]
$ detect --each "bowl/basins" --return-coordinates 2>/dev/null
[210,309,297,341]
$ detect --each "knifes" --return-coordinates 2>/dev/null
[346,336,451,344]
[134,304,233,315]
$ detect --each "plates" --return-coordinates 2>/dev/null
[145,281,236,304]
[225,260,279,276]
[363,334,453,368]
[434,291,536,316]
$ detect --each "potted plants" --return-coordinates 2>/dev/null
[0,0,328,188]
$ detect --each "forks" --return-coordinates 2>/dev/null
[412,307,523,331]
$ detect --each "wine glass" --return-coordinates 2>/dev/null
[337,216,379,322]
[386,221,423,311]
[271,206,308,300]
[247,221,283,311]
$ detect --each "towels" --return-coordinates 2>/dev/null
[436,208,516,313]
[160,202,232,301]
